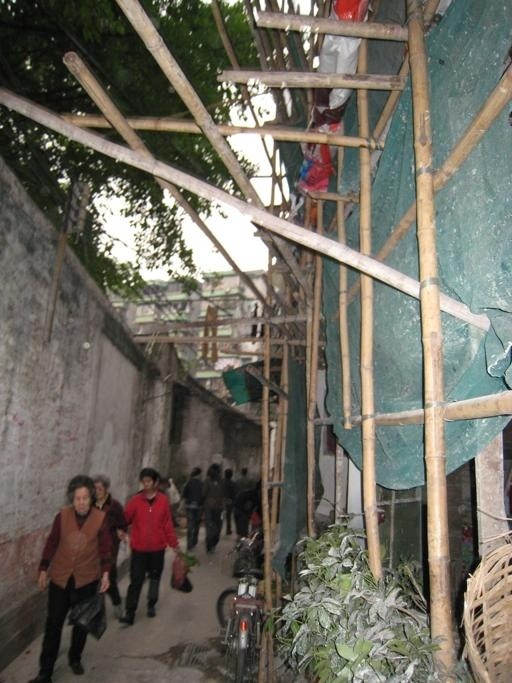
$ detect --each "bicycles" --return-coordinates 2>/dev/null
[216,527,263,683]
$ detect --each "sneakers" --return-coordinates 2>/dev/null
[110,602,156,627]
[27,672,55,683]
[67,659,84,678]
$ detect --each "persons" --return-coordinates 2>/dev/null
[87,474,127,619]
[27,474,114,682]
[157,463,274,554]
[116,467,179,626]
